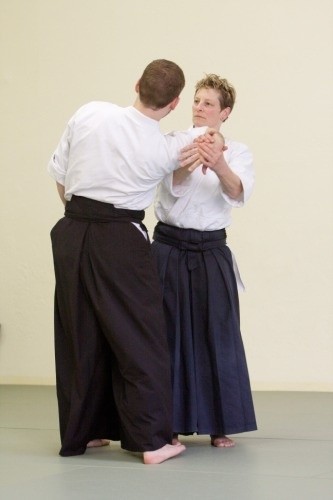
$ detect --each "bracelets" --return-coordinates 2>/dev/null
[212,130,225,148]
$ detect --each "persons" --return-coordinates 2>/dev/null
[50,57,227,464]
[142,73,258,451]
